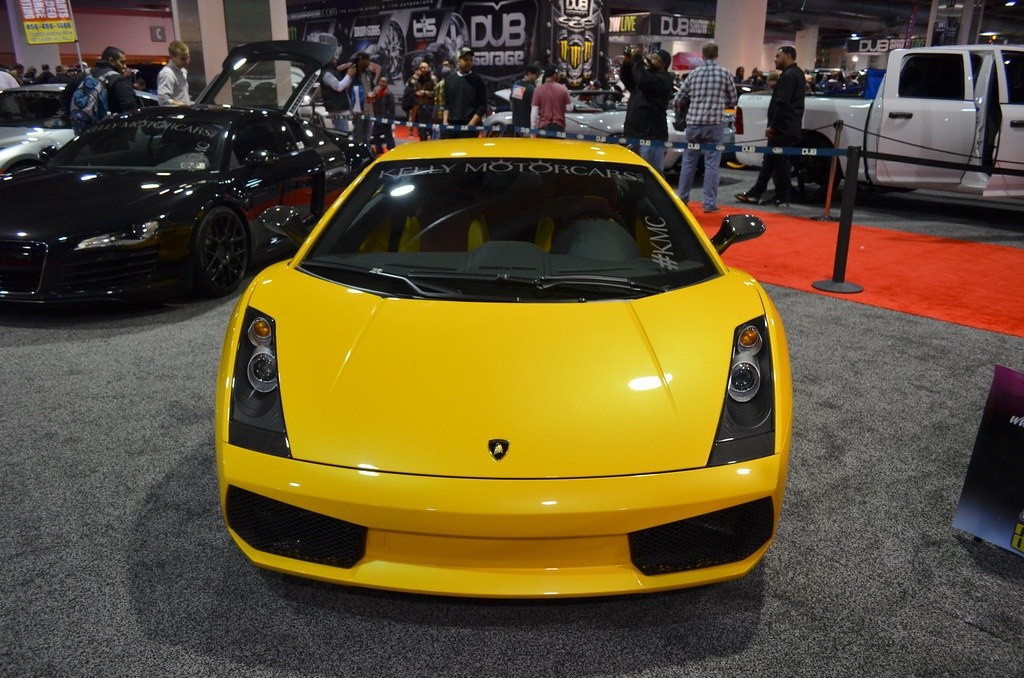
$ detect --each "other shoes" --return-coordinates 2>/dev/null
[734,192,759,204]
[760,194,791,207]
[703,207,720,212]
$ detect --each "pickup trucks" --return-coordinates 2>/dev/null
[733,30,1024,216]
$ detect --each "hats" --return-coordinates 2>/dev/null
[545,64,558,76]
[356,53,369,60]
[526,64,542,74]
[457,47,474,62]
[654,49,671,70]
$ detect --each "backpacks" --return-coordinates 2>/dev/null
[70,68,120,136]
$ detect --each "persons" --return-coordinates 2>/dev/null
[0,40,867,207]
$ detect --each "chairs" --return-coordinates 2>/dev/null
[533,179,617,255]
[398,180,491,254]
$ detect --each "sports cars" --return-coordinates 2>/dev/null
[213,133,796,605]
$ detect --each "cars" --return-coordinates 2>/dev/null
[0,38,376,322]
[481,89,727,178]
[813,67,846,91]
[1,83,165,178]
